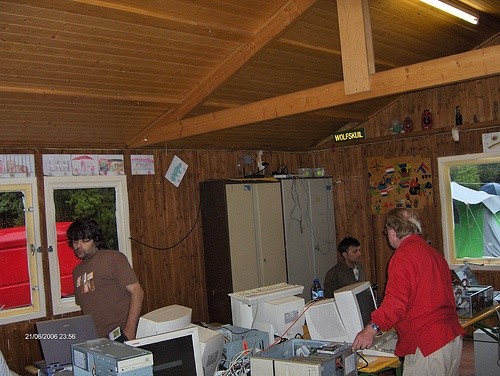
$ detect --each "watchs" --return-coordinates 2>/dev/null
[368,322,380,333]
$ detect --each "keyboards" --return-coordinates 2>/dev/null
[361,334,400,359]
[227,282,305,305]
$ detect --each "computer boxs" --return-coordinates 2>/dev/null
[448,284,494,318]
[212,323,269,368]
[246,340,358,376]
[69,337,153,376]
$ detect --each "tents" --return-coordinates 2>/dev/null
[451,182,500,259]
[481,183,500,196]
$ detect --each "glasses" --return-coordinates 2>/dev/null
[383,227,392,235]
[72,237,92,243]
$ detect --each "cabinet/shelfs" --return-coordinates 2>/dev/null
[199,175,338,327]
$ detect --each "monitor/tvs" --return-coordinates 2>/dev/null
[305,280,378,345]
[134,305,225,376]
[230,296,307,341]
[124,326,206,376]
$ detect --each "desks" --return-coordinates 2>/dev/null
[301,303,500,376]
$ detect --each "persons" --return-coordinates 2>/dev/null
[352,207,467,376]
[65,217,144,343]
[323,237,367,298]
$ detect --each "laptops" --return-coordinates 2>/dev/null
[30,314,100,374]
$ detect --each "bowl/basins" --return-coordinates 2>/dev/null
[296,168,325,177]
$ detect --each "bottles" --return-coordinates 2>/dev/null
[311,278,324,300]
[403,117,413,132]
[422,110,433,130]
[456,106,462,125]
